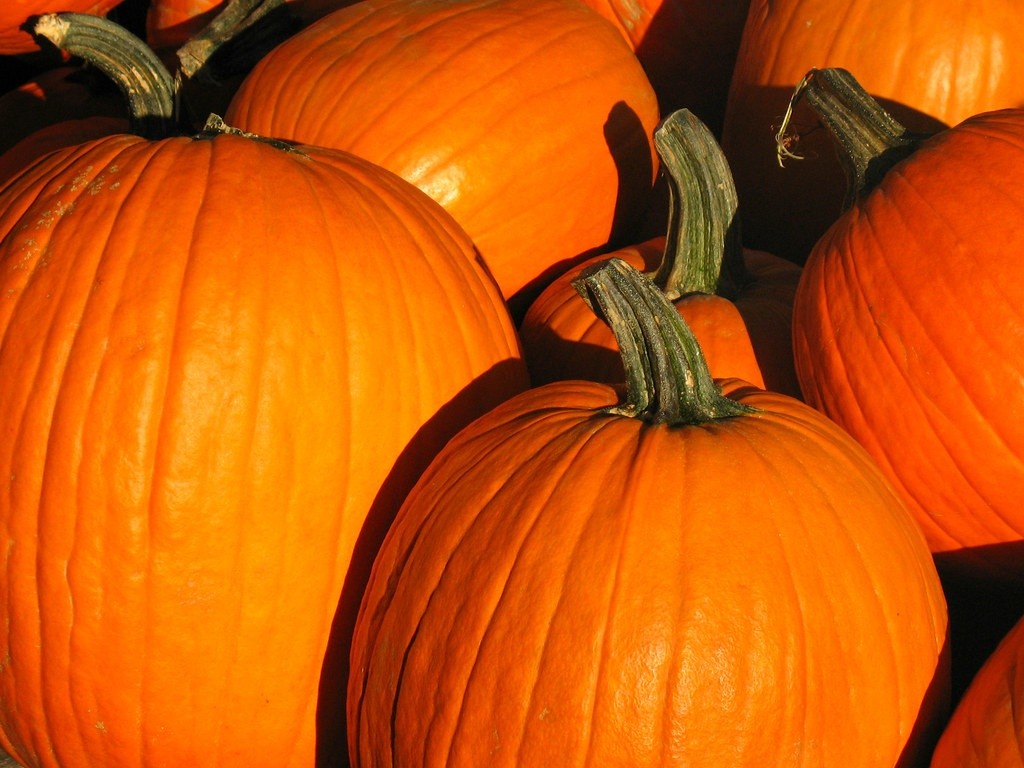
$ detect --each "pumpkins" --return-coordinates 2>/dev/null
[0,0,1024,768]
